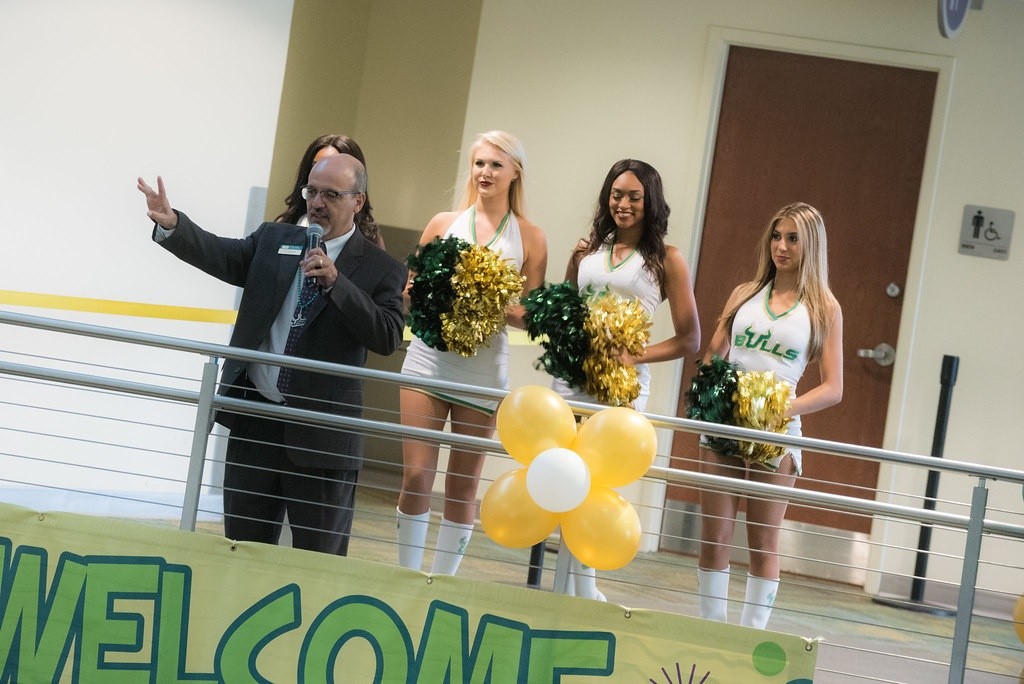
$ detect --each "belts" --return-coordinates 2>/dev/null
[245,379,288,407]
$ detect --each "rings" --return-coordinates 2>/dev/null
[320,260,324,268]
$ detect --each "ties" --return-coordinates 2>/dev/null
[276,242,327,400]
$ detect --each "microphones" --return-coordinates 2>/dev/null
[306,223,324,289]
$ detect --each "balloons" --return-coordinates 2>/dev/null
[479,386,655,571]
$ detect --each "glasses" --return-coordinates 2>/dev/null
[300,185,361,204]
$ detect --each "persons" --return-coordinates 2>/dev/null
[137,134,409,556]
[396,130,548,576]
[697,202,845,630]
[552,158,701,601]
